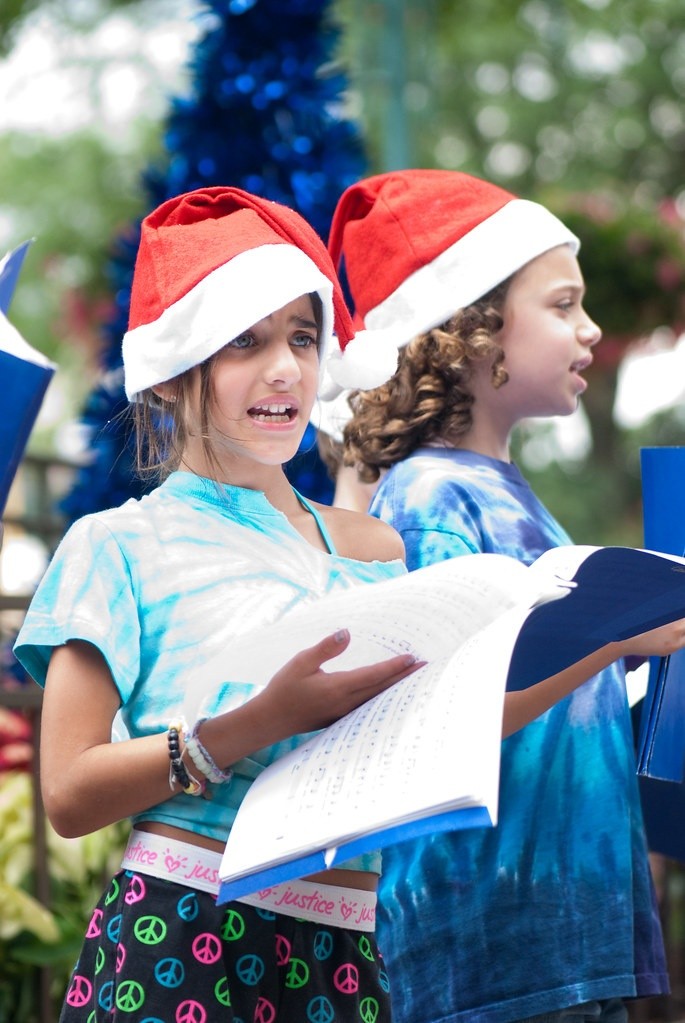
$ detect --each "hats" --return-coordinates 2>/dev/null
[123,186,400,409]
[319,169,577,403]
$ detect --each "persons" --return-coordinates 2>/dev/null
[15,184,429,1022]
[327,169,669,1023]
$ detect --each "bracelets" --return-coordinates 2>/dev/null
[168,721,205,797]
[186,719,233,783]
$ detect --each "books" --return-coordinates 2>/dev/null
[216,545,685,905]
[637,445,685,784]
